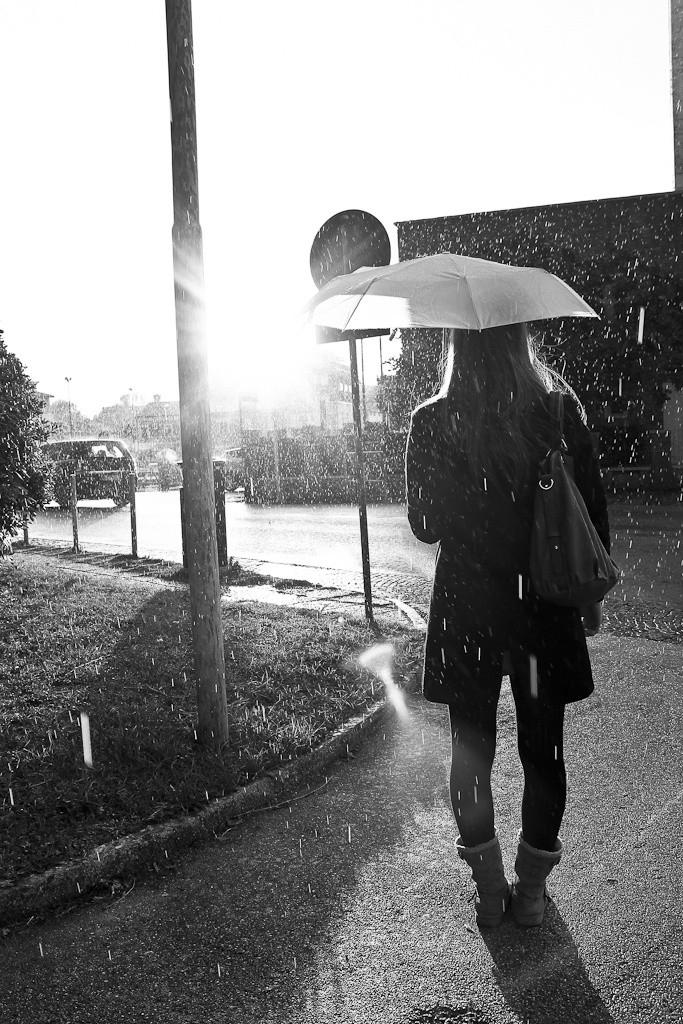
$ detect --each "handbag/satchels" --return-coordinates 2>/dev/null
[527,390,622,610]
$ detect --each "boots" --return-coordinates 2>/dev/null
[454,826,513,928]
[511,828,565,927]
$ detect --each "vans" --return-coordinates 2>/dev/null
[38,439,137,508]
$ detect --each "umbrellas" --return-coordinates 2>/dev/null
[302,251,604,339]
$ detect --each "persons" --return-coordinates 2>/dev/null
[406,317,611,929]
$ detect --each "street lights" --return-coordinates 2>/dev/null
[64,376,73,439]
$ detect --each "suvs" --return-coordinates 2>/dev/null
[216,448,245,491]
